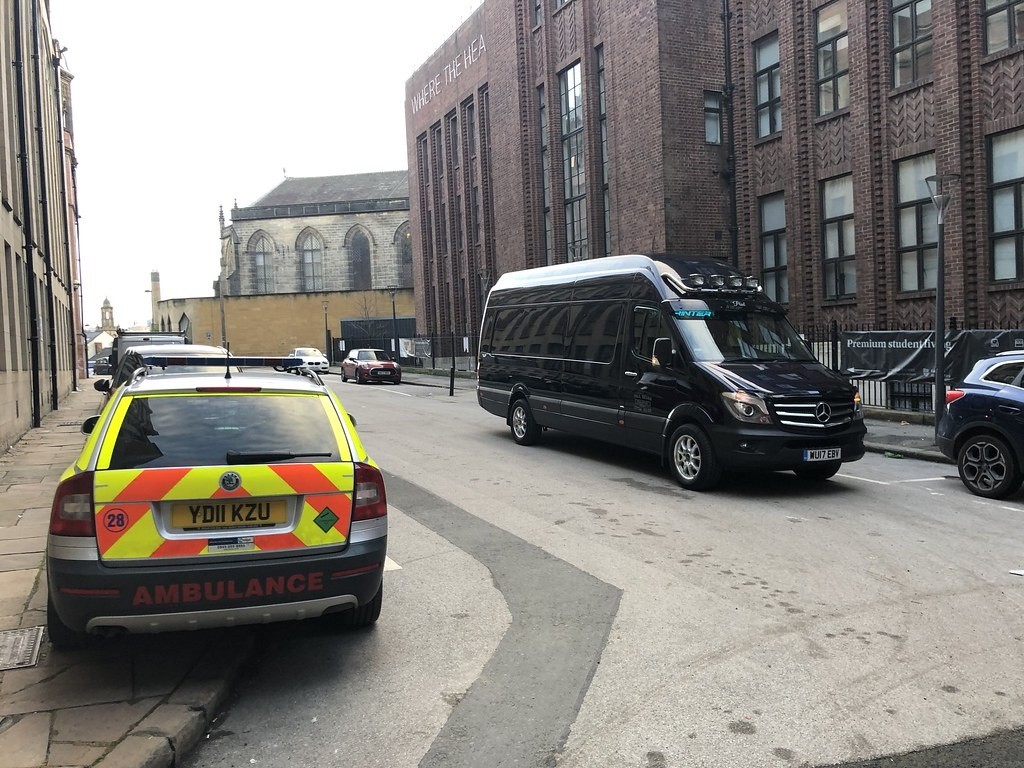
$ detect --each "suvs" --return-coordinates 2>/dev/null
[936,351,1024,500]
[93,345,244,414]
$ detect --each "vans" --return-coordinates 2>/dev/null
[474,251,869,492]
[108,328,189,378]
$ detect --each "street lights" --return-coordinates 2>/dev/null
[386,284,400,364]
[322,300,331,354]
[923,171,964,433]
[478,268,491,306]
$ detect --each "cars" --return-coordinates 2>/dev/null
[289,347,330,375]
[340,348,402,385]
[87,357,113,375]
[42,354,389,653]
